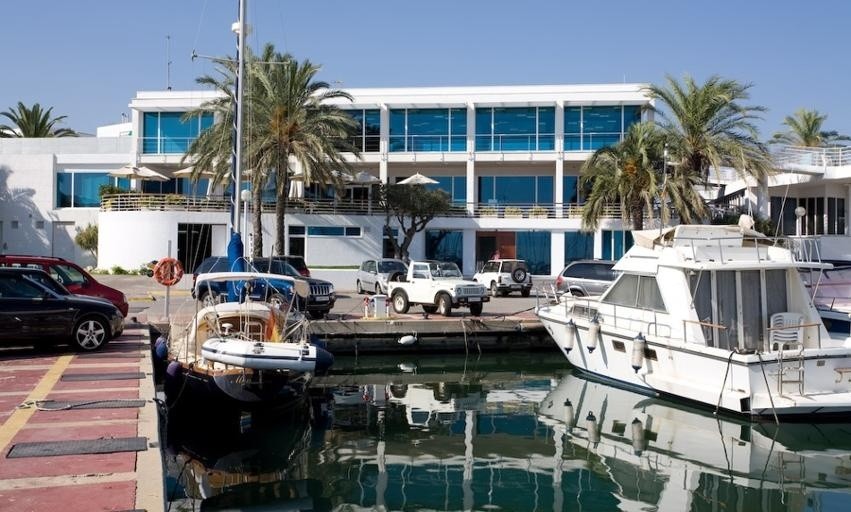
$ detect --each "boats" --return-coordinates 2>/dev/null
[161,392,330,512]
[526,373,850,500]
[532,140,851,424]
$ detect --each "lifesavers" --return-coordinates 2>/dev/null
[154,258,184,286]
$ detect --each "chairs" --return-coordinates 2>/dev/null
[768,310,804,351]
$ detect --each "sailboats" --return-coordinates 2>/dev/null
[163,0,340,417]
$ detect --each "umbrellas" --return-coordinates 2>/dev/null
[173,164,382,209]
[397,171,441,187]
[132,166,170,193]
[104,162,148,190]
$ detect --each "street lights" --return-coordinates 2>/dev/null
[238,187,255,257]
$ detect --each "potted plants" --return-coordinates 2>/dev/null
[104,194,304,214]
[479,206,549,220]
[567,204,613,219]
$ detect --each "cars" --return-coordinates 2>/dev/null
[353,256,409,297]
[268,254,312,278]
[0,253,131,357]
[555,259,622,300]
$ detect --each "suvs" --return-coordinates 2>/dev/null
[471,257,534,299]
[191,253,338,320]
[384,258,493,319]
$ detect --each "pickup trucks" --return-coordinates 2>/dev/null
[386,381,492,434]
